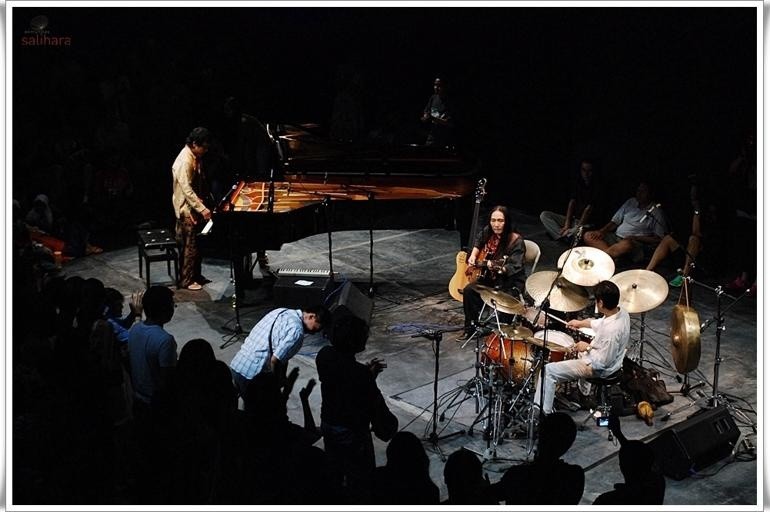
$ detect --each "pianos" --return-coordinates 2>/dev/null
[195,122,476,309]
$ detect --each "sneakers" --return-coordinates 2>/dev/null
[669,276,684,287]
[457,333,477,342]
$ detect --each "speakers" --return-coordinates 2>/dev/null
[663,405,741,473]
[326,281,377,350]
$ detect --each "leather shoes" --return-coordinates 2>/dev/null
[188,282,202,290]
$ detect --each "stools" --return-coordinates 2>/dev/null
[583,375,619,425]
[137,218,178,289]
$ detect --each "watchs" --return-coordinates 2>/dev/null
[586,344,592,354]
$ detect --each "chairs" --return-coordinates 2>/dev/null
[513,240,541,325]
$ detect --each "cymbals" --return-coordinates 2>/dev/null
[558,246,615,286]
[494,325,533,342]
[609,270,669,313]
[526,271,589,313]
[480,289,525,315]
[528,339,569,354]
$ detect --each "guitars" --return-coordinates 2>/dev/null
[465,248,508,284]
[448,178,487,302]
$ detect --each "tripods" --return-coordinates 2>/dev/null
[461,361,526,467]
[214,209,253,350]
[411,326,465,462]
[645,210,711,401]
[661,270,754,455]
[439,309,496,432]
[468,318,530,460]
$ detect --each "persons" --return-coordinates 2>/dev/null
[523,279,631,425]
[171,127,212,291]
[408,411,670,505]
[582,179,666,268]
[539,158,608,246]
[644,178,739,288]
[455,205,527,343]
[13,188,407,504]
[419,76,456,145]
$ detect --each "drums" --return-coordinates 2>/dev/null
[481,331,544,388]
[536,328,576,362]
